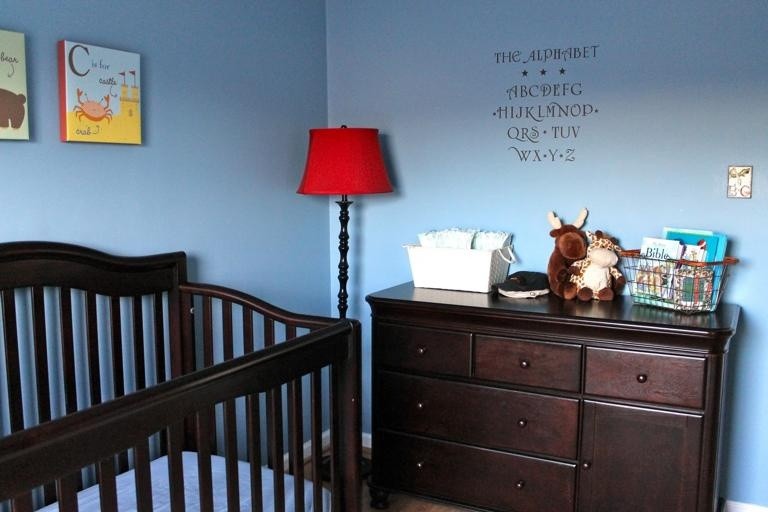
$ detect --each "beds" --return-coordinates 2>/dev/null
[0,241,363,512]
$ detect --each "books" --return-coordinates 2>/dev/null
[632,225,727,313]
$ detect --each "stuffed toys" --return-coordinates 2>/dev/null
[544,208,588,301]
[566,229,625,302]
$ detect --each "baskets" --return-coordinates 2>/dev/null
[611,244,740,313]
[402,241,518,296]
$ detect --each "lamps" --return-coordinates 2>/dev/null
[296,124,394,317]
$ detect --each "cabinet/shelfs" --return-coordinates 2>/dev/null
[364,282,742,512]
